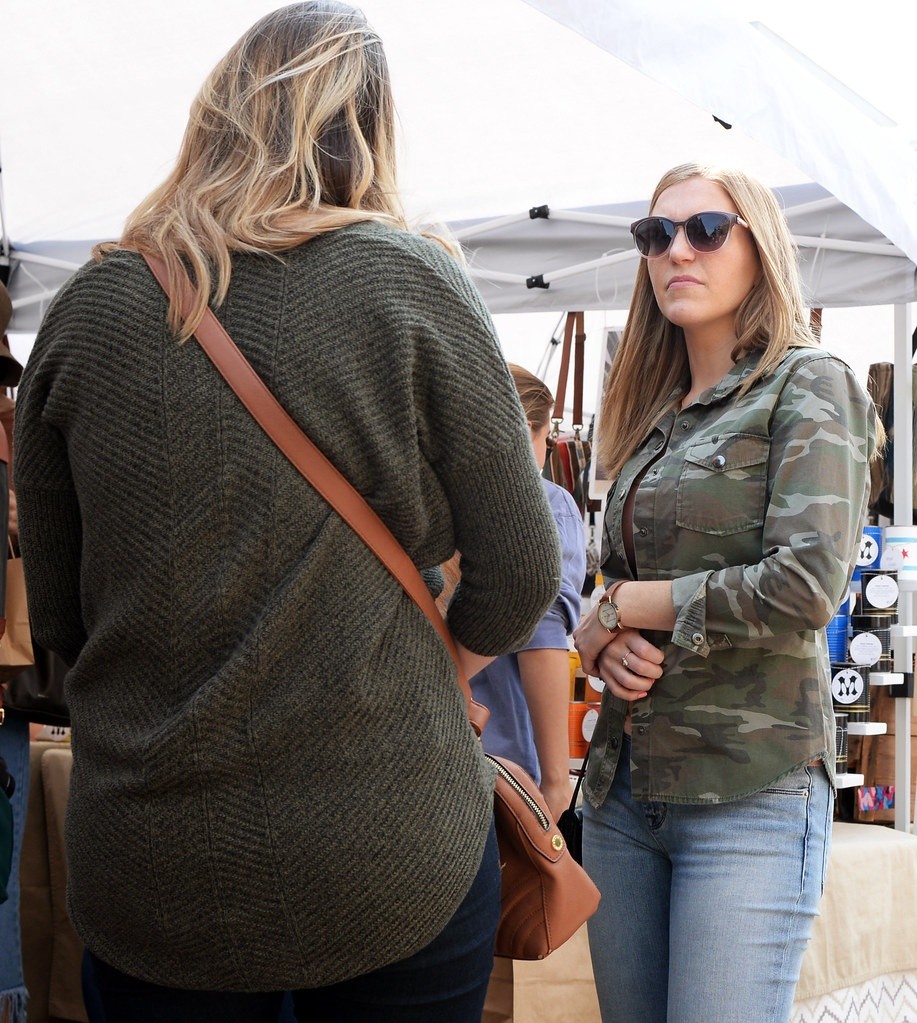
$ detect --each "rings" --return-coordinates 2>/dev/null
[621,651,634,667]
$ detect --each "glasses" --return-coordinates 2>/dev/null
[630,209,750,261]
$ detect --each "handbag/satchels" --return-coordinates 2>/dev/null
[484,754,602,966]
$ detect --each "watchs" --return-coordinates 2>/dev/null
[598,579,630,634]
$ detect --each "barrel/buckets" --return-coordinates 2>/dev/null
[825,614,850,661]
[885,524,917,581]
[851,612,892,673]
[833,583,851,623]
[831,712,850,773]
[830,661,872,722]
[852,525,882,581]
[859,567,899,626]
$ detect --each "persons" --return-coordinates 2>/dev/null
[14,1,560,1022]
[570,162,879,1023]
[467,364,587,825]
